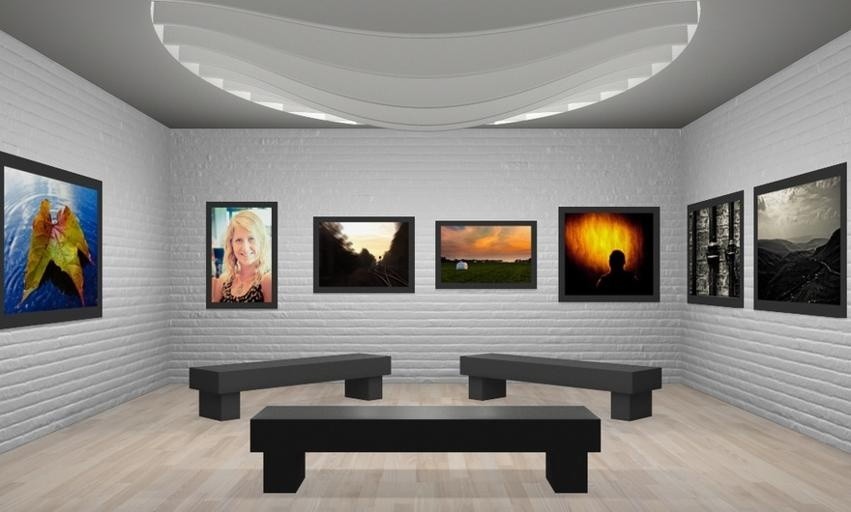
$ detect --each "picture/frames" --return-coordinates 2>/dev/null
[557,205,661,303]
[753,162,846,318]
[435,220,537,290]
[312,215,415,294]
[205,201,279,310]
[0,150,103,329]
[686,189,744,310]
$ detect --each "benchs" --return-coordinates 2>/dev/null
[188,353,392,422]
[458,352,661,422]
[246,405,601,495]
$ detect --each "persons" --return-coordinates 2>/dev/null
[594,250,641,294]
[211,210,273,304]
